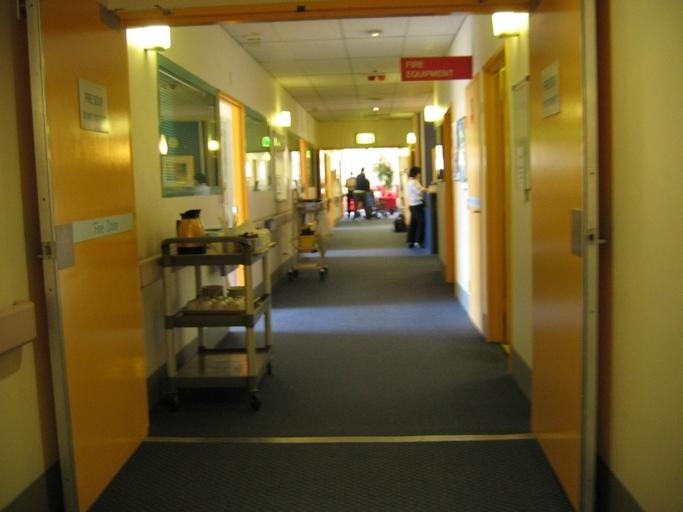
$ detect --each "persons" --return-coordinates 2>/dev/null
[405,166,432,248]
[345,167,369,219]
[192,172,211,195]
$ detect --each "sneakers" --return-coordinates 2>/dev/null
[408,241,425,249]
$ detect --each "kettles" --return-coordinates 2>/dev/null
[175,208,205,254]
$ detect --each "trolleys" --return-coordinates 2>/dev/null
[286,199,329,281]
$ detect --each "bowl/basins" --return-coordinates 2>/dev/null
[185,295,248,312]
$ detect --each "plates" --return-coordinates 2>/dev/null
[196,285,247,296]
